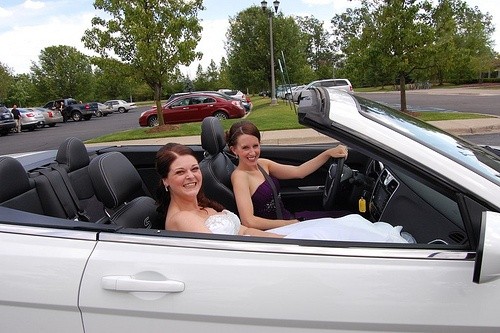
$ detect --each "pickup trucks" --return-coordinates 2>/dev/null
[43,98,98,122]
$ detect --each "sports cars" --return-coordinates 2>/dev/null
[0,85,500,333]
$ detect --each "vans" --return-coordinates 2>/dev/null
[294,78,354,105]
[166,91,250,114]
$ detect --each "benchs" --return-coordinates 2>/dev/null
[0,137,111,225]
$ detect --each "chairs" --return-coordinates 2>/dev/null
[88,151,159,230]
[198,116,239,218]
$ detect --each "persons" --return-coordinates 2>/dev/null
[156,143,418,243]
[228,121,348,229]
[79,101,82,104]
[11,104,22,132]
[58,101,67,123]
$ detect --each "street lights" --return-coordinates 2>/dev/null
[261,0,280,105]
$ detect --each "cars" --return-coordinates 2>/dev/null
[259,84,306,100]
[86,103,114,117]
[36,107,63,128]
[138,93,248,127]
[104,100,138,113]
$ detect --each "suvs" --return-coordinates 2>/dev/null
[218,89,245,102]
[0,104,46,136]
